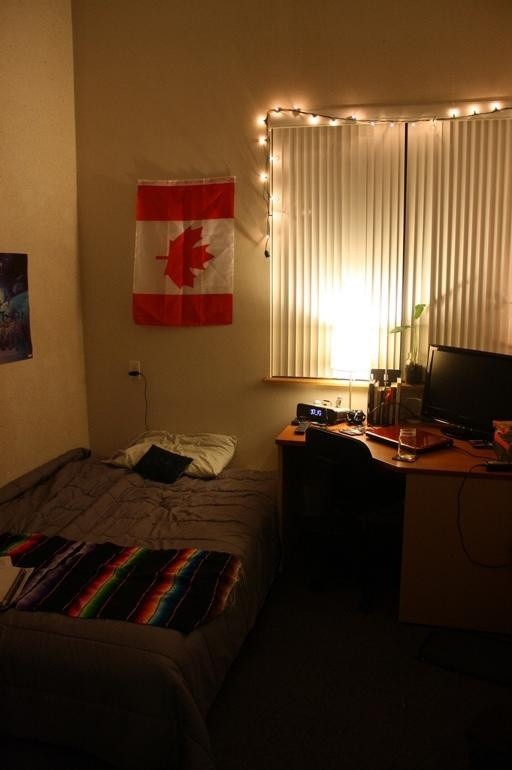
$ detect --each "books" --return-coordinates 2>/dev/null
[367,383,402,425]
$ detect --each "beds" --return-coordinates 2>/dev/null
[0,447,276,770]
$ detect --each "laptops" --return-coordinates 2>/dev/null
[365,423,453,456]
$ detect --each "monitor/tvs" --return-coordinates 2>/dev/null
[419,343,512,441]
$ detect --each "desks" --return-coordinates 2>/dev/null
[273,409,512,637]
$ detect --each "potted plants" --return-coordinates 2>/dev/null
[387,301,428,384]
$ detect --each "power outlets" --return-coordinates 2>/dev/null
[128,359,142,380]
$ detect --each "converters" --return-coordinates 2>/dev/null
[485,458,512,471]
[128,370,140,376]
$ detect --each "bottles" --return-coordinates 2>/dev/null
[398,427,417,460]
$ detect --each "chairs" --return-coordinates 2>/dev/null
[301,423,394,632]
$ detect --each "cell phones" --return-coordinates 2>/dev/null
[295,421,308,433]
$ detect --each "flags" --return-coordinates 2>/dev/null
[131,176,237,327]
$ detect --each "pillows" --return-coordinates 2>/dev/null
[102,429,241,479]
[128,443,193,484]
